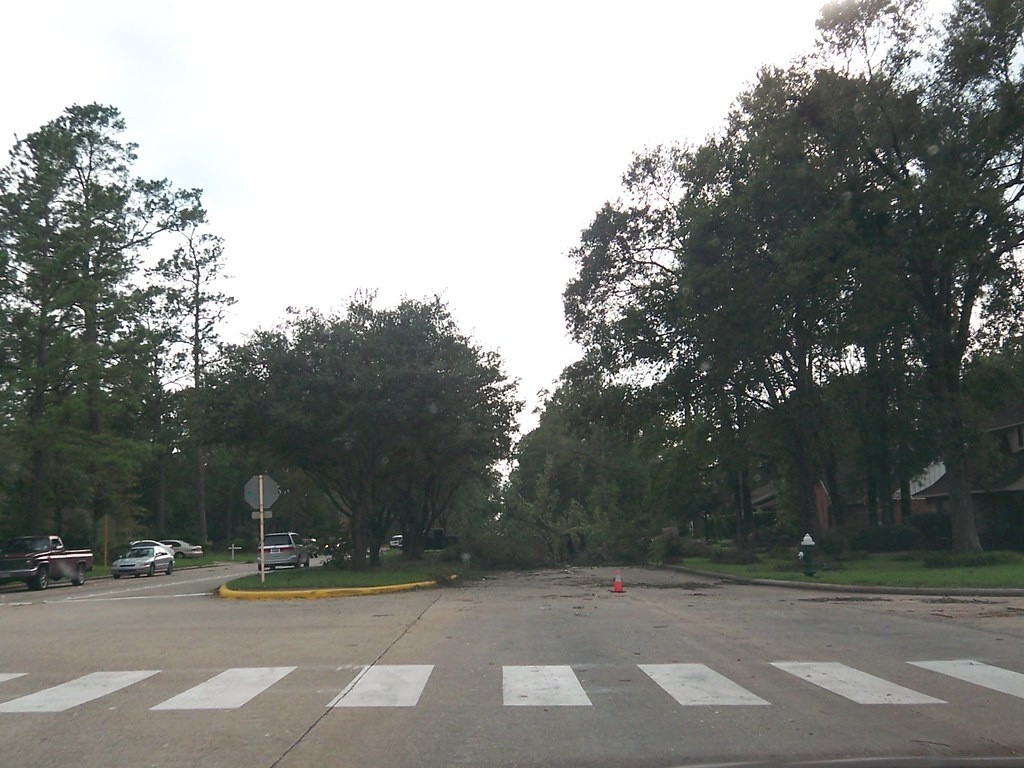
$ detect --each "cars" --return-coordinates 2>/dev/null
[119,540,176,558]
[161,540,205,559]
[111,548,175,578]
[302,536,348,559]
[257,532,310,569]
[390,535,403,548]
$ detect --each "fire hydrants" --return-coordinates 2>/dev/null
[798,533,822,578]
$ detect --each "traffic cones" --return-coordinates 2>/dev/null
[609,569,626,593]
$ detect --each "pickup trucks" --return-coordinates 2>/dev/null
[0,535,94,591]
